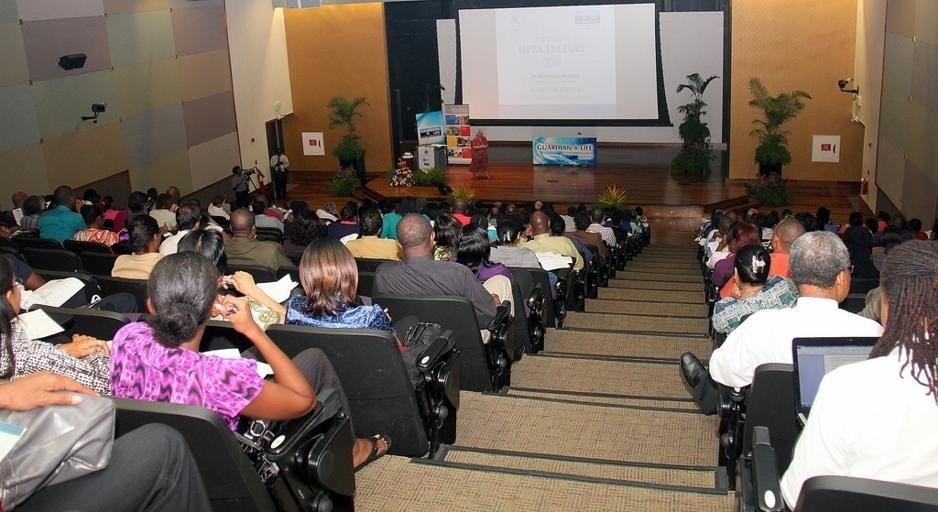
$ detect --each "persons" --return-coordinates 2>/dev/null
[106,248,393,479]
[0,368,214,511]
[0,254,112,399]
[695,206,938,347]
[3,172,652,343]
[270,147,290,200]
[677,232,887,416]
[762,237,937,512]
[230,165,253,206]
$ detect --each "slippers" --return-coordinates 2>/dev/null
[354,432,392,473]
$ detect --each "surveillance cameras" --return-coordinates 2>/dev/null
[92,105,106,112]
[838,77,853,87]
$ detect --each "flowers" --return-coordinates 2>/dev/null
[332,164,358,185]
[752,171,788,195]
[390,155,414,187]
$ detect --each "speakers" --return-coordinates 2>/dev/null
[58,53,87,70]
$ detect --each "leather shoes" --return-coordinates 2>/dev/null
[680,352,706,389]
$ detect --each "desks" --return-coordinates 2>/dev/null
[531,133,600,168]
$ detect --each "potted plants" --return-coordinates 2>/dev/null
[742,74,814,182]
[329,93,372,180]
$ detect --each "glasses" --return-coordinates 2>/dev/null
[841,265,855,274]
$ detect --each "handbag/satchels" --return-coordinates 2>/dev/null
[398,320,444,392]
[232,418,280,484]
[0,389,116,512]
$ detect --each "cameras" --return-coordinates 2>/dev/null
[242,168,255,176]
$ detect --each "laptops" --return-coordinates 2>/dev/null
[792,337,883,428]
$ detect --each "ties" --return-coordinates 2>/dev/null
[278,157,280,173]
